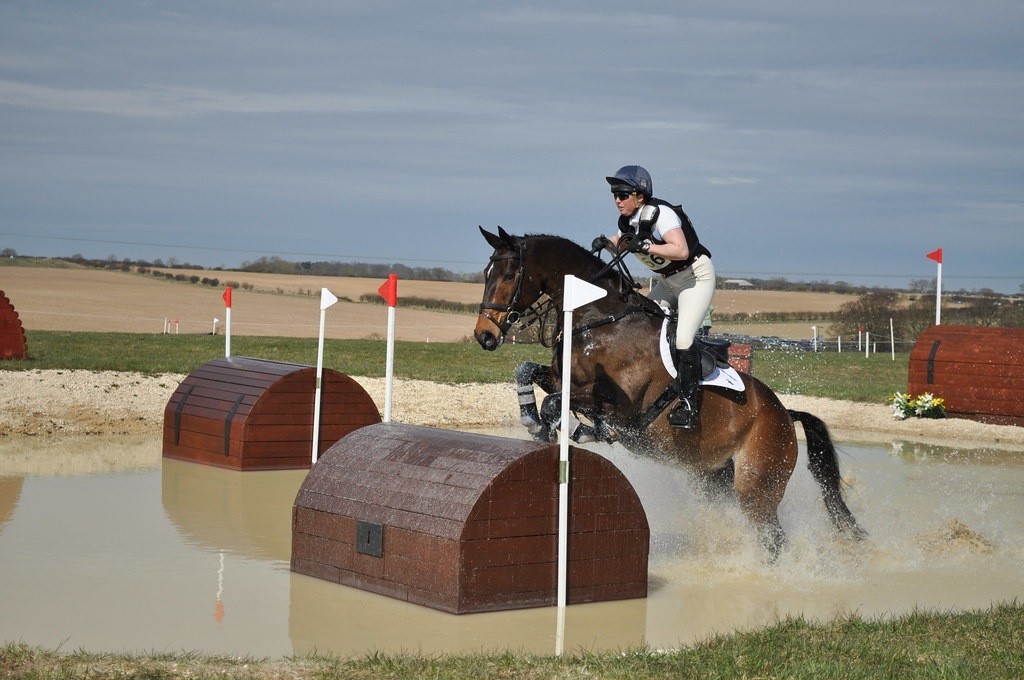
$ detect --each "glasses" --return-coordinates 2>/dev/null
[614,192,633,200]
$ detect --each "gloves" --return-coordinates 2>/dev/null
[592,237,608,251]
[627,238,650,255]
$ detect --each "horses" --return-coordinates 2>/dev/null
[474,223,870,564]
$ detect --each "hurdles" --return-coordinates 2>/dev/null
[162,353,383,472]
[908,326,1024,428]
[289,422,651,617]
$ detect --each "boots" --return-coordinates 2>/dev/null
[668,340,701,425]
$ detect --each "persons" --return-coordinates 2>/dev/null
[592,166,717,426]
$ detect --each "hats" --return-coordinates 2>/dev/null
[606,164,652,195]
